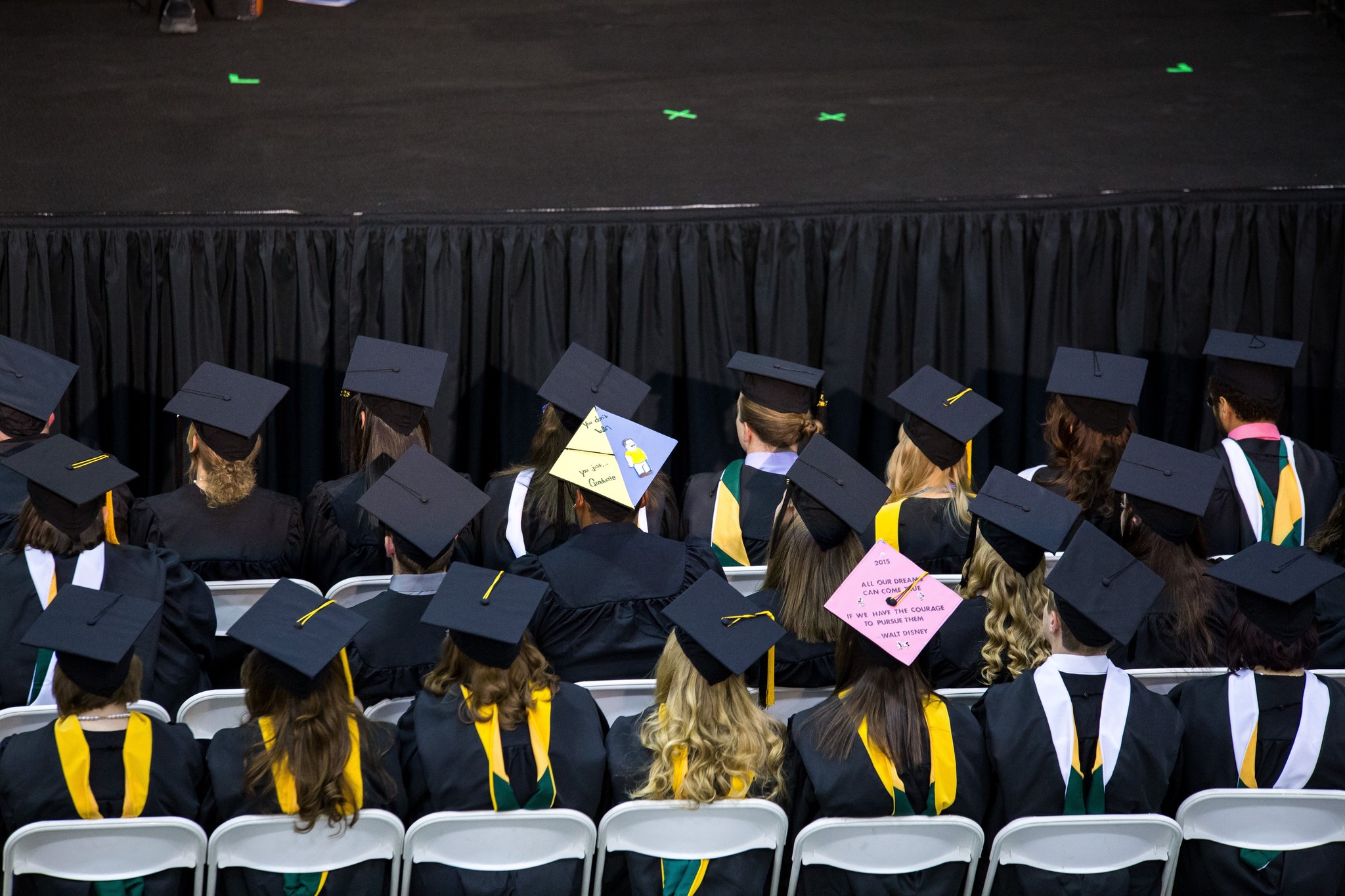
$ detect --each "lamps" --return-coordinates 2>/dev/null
[158,0,198,34]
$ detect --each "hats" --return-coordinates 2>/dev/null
[547,406,679,523]
[420,560,549,670]
[1110,431,1224,545]
[0,334,79,436]
[1202,540,1344,636]
[537,342,650,437]
[342,335,449,437]
[660,568,788,687]
[21,583,162,699]
[163,361,290,463]
[823,540,964,667]
[786,432,893,552]
[1045,346,1149,437]
[966,465,1084,578]
[727,349,824,414]
[1202,327,1304,402]
[1,433,139,541]
[355,443,491,572]
[887,364,1005,473]
[1042,520,1164,647]
[225,576,370,676]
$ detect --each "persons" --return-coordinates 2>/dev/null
[680,352,827,567]
[1189,539,1345,896]
[344,443,492,704]
[914,466,1083,687]
[413,562,608,896]
[791,541,986,896]
[1098,432,1238,669]
[1016,347,1150,508]
[480,343,680,569]
[0,434,168,707]
[974,522,1185,896]
[303,336,473,585]
[609,574,789,896]
[0,585,203,896]
[1306,488,1345,557]
[131,360,305,576]
[1199,327,1338,560]
[746,435,894,689]
[204,576,409,896]
[504,407,730,678]
[0,334,80,553]
[858,363,1007,575]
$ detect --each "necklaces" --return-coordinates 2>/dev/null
[77,712,130,721]
[1254,672,1299,676]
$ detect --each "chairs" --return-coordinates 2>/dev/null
[0,554,1345,896]
